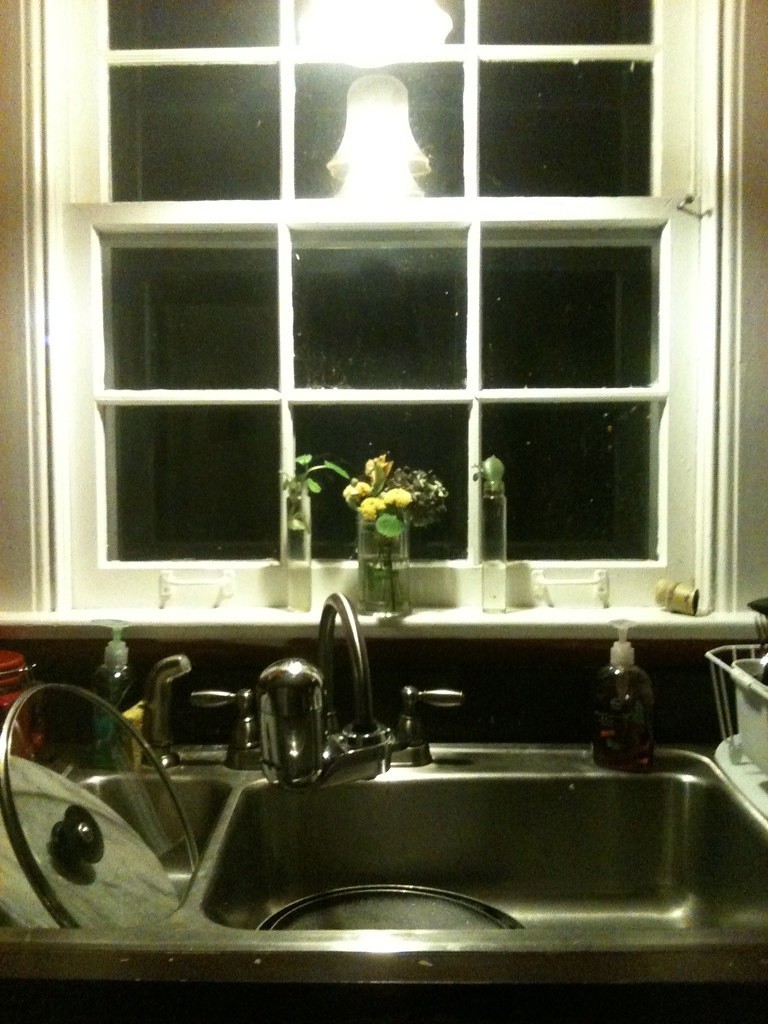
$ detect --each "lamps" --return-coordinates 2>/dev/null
[329,74,432,201]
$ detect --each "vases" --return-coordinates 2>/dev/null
[479,482,507,614]
[356,513,413,616]
[286,480,312,612]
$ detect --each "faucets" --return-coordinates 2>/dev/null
[256,590,390,794]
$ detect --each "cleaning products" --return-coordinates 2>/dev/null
[82,617,134,771]
[590,617,656,771]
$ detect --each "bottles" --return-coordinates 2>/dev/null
[0,650,37,761]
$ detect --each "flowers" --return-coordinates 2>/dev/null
[342,453,449,538]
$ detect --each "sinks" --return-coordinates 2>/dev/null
[0,744,240,981]
[178,745,768,988]
